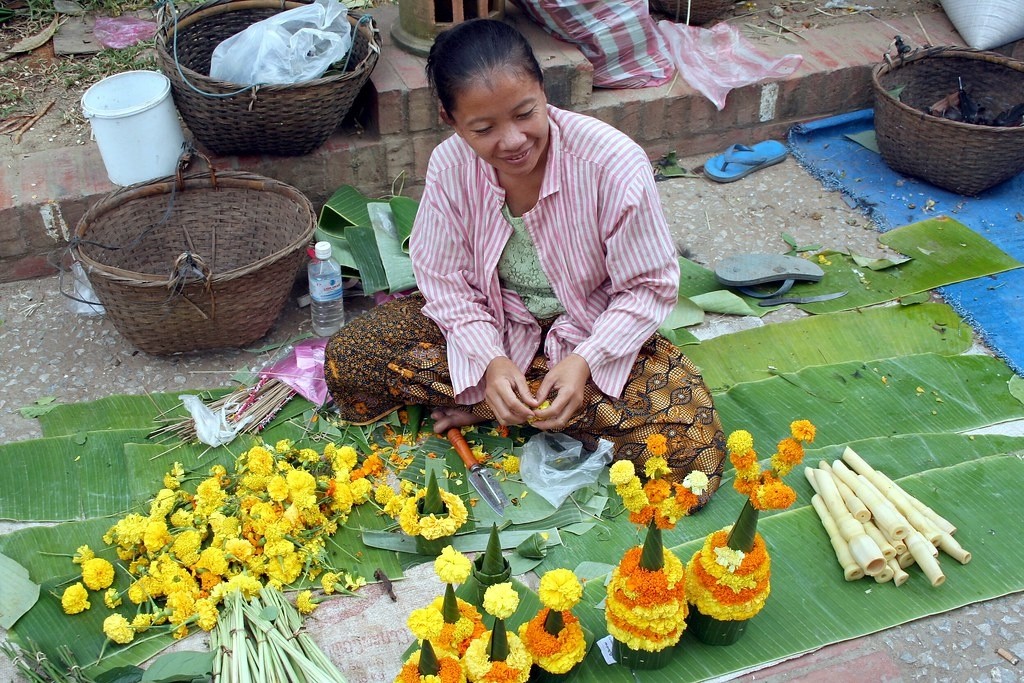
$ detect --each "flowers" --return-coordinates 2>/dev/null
[38,420,818,683]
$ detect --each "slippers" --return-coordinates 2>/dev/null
[704,139,788,183]
[717,253,826,299]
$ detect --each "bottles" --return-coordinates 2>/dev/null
[307,240,348,337]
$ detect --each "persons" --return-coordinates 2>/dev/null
[320,18,725,515]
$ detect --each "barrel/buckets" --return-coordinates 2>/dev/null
[78,67,193,189]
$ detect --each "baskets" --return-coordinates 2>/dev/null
[155,0,382,154]
[872,37,1023,197]
[71,149,317,355]
[646,0,737,26]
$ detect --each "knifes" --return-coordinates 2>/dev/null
[448,428,509,517]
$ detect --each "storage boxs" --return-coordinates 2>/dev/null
[870,35,1024,197]
[71,150,319,356]
[156,0,383,157]
